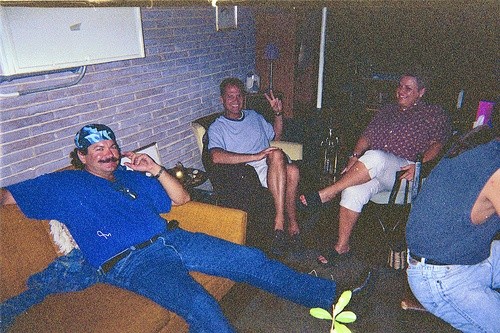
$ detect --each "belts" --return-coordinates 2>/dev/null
[409,250,450,266]
[96,219,179,273]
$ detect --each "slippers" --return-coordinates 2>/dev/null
[316,245,352,267]
[295,191,324,212]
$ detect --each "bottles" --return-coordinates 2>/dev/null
[252,75,258,93]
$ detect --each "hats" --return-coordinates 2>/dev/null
[74,124,115,149]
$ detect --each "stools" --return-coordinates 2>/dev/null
[399,266,453,333]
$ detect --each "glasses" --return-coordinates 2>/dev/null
[110,181,137,200]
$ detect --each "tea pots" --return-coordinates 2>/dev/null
[171,161,185,180]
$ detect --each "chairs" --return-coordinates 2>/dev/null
[368,136,454,265]
[190,112,304,209]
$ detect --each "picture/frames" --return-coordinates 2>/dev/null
[215,5,238,31]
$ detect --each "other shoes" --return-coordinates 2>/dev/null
[336,269,378,311]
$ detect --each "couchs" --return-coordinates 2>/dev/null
[0,143,248,333]
